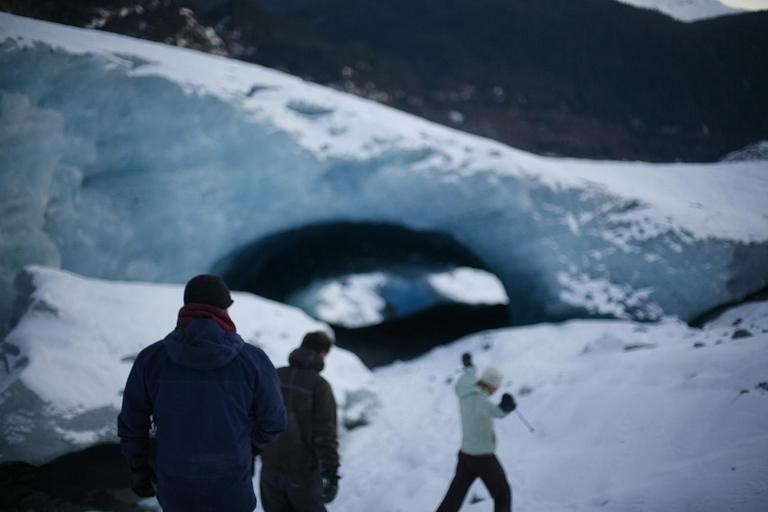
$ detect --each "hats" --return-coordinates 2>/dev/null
[184,274,233,308]
[479,365,503,389]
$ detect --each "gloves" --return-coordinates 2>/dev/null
[125,453,157,498]
[499,394,516,412]
[320,474,338,502]
[462,353,470,366]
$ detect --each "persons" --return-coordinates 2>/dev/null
[117,274,288,512]
[435,353,517,512]
[252,332,342,512]
